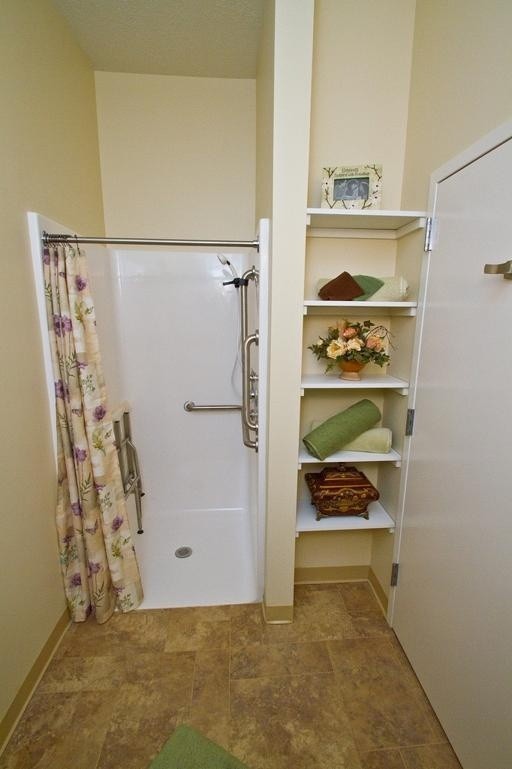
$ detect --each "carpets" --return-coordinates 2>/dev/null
[143,724,257,769]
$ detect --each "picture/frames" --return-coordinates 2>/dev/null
[320,163,383,211]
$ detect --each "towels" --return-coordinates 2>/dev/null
[302,398,383,461]
[319,272,364,300]
[310,420,392,454]
[315,277,409,301]
[347,274,384,300]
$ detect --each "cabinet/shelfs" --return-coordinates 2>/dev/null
[292,208,432,539]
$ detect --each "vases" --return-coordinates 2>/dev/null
[335,358,366,381]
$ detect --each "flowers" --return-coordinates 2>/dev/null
[307,318,399,374]
